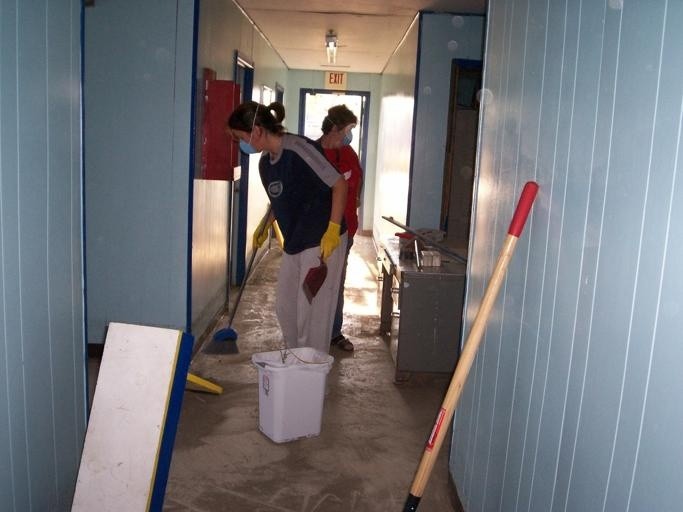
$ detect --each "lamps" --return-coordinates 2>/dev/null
[324,28,338,65]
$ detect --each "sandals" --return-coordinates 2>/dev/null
[333,334,353,351]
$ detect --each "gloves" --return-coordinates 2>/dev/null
[319,221,342,259]
[252,214,273,247]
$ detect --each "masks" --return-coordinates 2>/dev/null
[240,125,259,154]
[333,124,352,144]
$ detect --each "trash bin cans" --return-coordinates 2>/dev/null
[252,347,334,444]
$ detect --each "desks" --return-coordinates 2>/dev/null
[376,237,466,375]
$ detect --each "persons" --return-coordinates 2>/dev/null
[223,97,351,355]
[317,104,364,354]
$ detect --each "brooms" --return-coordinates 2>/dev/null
[201,202,274,354]
